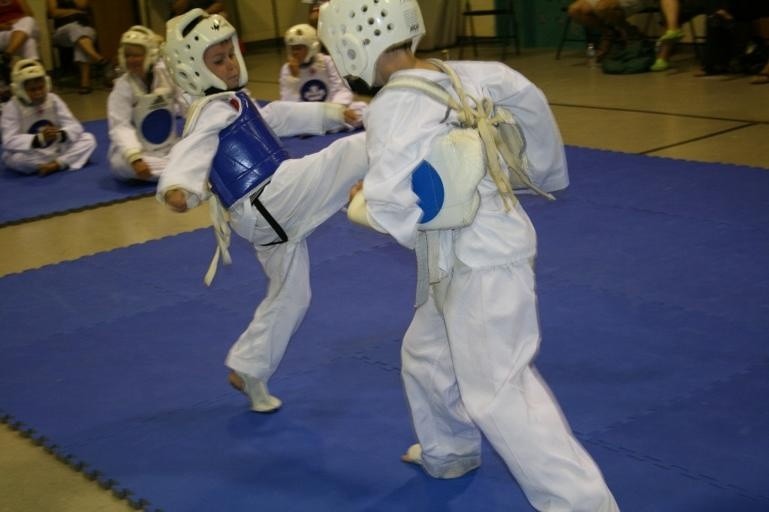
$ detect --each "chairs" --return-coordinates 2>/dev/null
[41,5,106,92]
[453,0,712,68]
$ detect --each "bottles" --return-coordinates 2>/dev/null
[441,48,450,60]
[586,43,597,71]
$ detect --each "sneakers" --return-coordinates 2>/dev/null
[656,30,687,48]
[650,54,674,73]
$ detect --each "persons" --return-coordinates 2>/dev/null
[274,20,358,136]
[566,0,769,85]
[156,7,369,415]
[316,0,621,511]
[170,0,232,22]
[47,1,104,95]
[105,25,191,183]
[1,1,41,77]
[0,58,97,175]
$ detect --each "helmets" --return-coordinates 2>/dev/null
[9,58,53,106]
[157,7,254,97]
[284,22,321,65]
[117,24,163,75]
[316,0,429,88]
